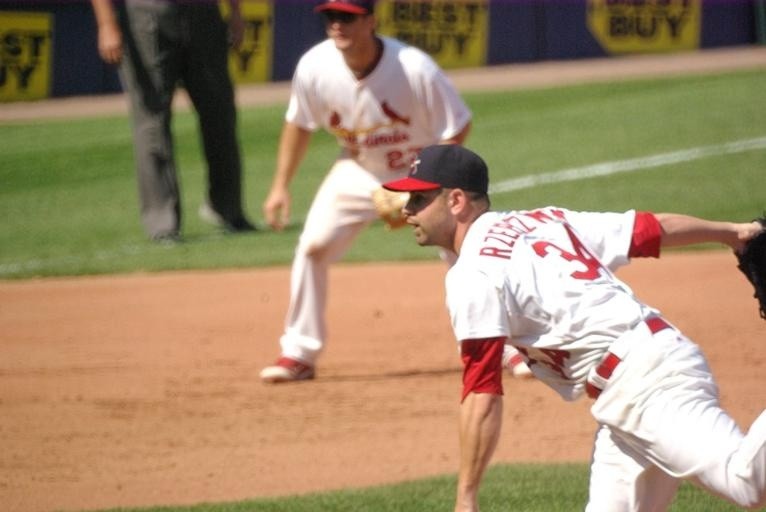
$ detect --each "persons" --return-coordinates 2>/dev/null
[257,1,537,385]
[88,0,280,248]
[379,140,766,511]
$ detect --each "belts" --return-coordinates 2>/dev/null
[585,317,671,400]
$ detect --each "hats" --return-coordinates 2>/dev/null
[382,144,488,194]
[312,0,375,17]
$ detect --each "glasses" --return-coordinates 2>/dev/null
[327,9,356,24]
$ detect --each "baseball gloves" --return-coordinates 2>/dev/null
[376,186,409,229]
[735,216,766,319]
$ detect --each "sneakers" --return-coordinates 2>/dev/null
[502,345,532,377]
[259,356,314,381]
